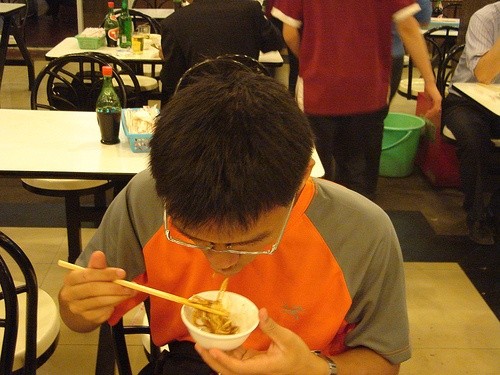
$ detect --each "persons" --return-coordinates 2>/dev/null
[440,1,500,245]
[58,71,413,375]
[388,0,433,105]
[261,0,300,101]
[160,0,284,109]
[270,0,443,201]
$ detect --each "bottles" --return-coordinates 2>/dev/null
[104,2,119,47]
[117,0,132,48]
[95,66,122,123]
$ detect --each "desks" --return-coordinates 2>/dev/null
[0,2,38,92]
[0,107,324,268]
[451,82,500,117]
[402,17,460,78]
[111,8,174,33]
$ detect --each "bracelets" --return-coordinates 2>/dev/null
[309,350,337,375]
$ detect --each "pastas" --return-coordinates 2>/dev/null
[189,278,239,335]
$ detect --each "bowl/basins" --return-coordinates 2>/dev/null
[180,290,260,352]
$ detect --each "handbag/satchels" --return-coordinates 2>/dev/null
[51,70,143,111]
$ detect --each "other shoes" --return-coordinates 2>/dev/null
[468,226,496,245]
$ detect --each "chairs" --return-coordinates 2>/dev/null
[0,0,500,375]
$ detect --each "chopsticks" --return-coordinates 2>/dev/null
[124,107,159,134]
[57,260,231,317]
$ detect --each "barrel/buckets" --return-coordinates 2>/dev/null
[378,112,425,178]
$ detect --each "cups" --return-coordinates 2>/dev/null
[96,106,121,145]
[131,32,145,53]
[138,25,150,40]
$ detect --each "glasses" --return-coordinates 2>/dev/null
[162,184,298,256]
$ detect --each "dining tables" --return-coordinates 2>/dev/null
[44,33,284,94]
